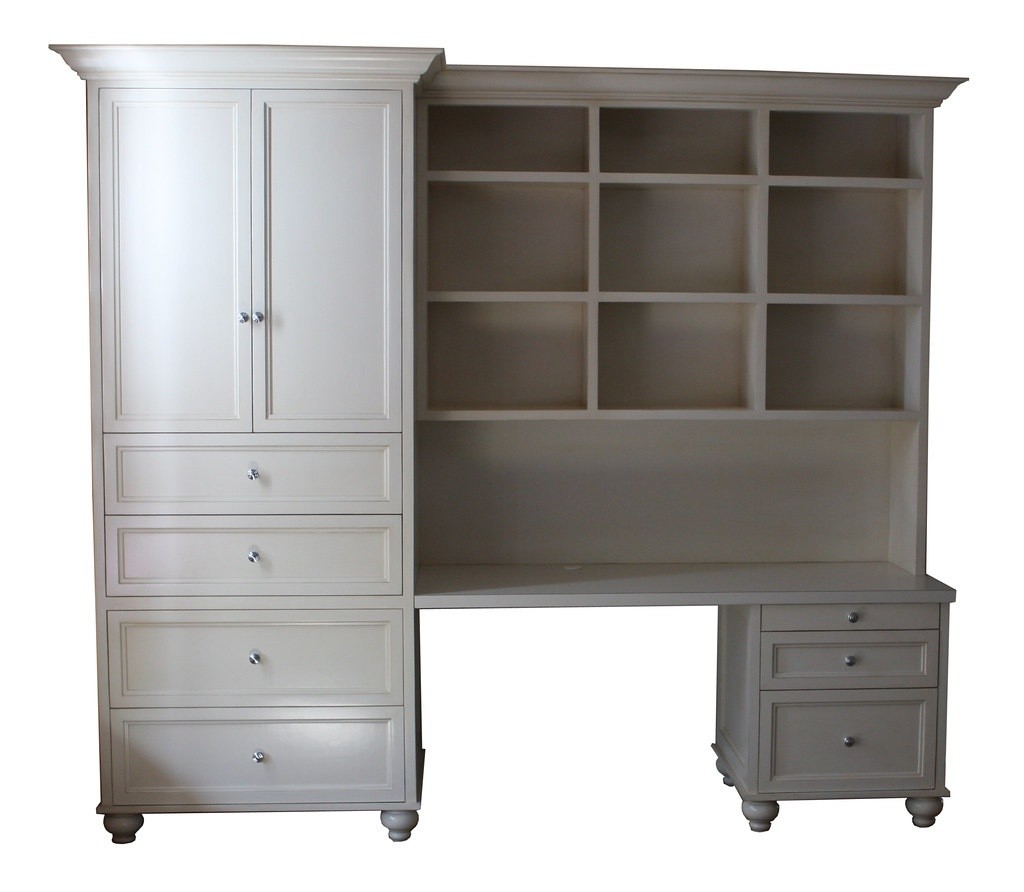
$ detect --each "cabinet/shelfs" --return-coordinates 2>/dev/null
[49,43,969,845]
